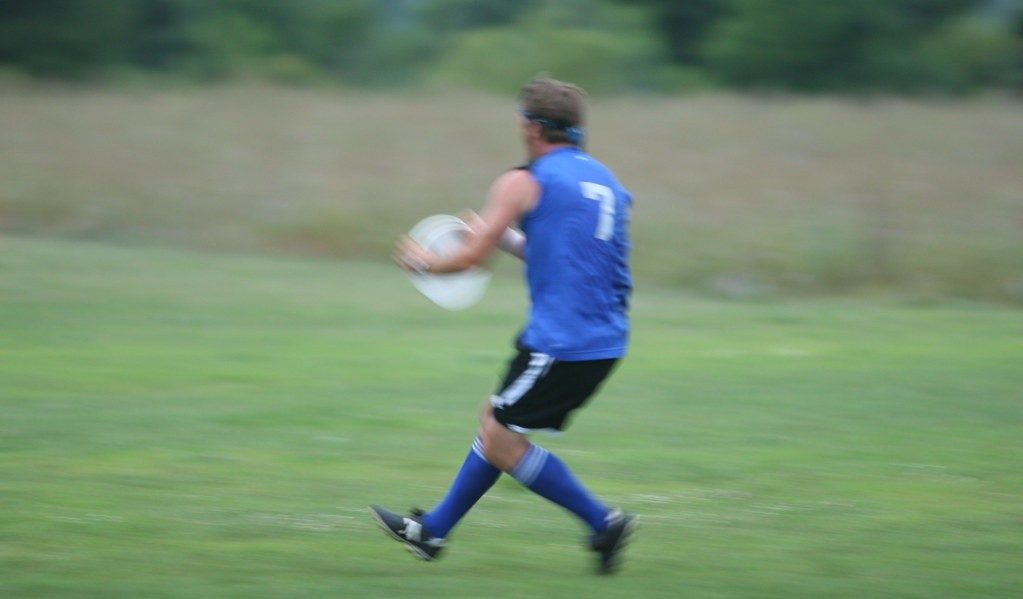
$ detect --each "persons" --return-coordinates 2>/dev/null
[370,77,638,578]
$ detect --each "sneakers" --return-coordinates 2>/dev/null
[366,504,445,563]
[589,508,638,575]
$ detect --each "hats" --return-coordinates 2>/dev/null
[527,80,589,128]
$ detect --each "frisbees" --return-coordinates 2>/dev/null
[408,211,491,310]
